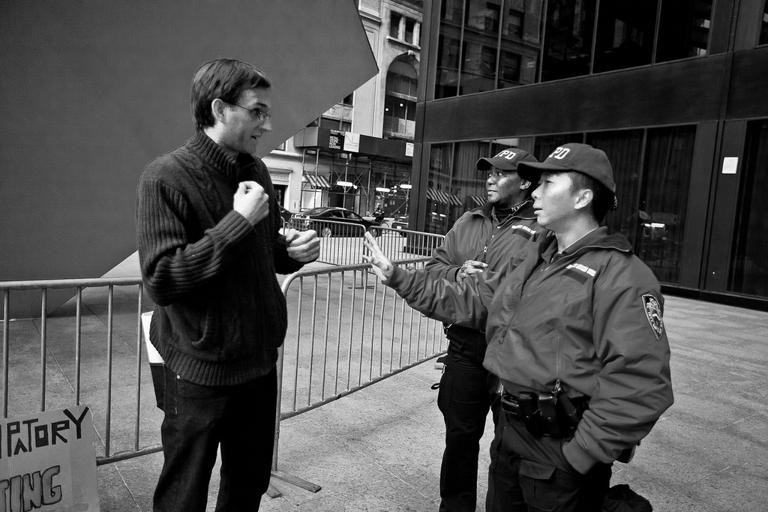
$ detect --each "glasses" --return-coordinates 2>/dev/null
[234,103,272,121]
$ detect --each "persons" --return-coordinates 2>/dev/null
[135,56,321,512]
[422,147,553,512]
[361,142,675,509]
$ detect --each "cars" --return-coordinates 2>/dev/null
[393,210,446,237]
[291,207,381,238]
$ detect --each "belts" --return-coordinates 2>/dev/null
[497,392,538,422]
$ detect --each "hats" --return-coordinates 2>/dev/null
[477,147,538,172]
[515,142,617,195]
